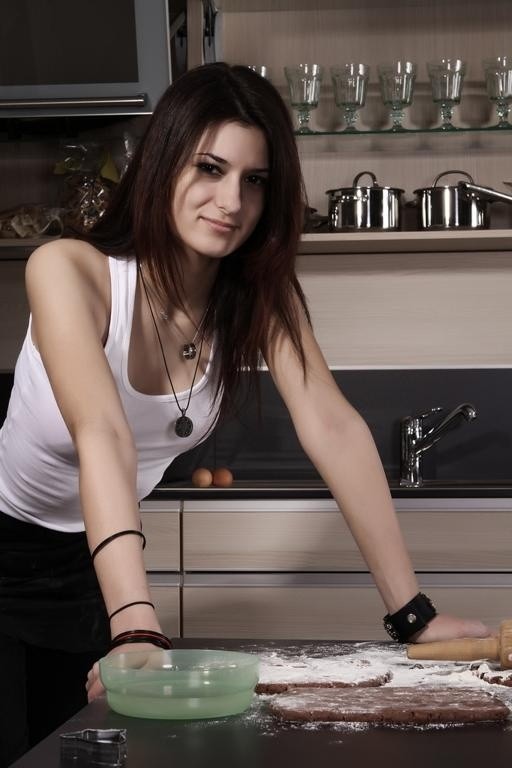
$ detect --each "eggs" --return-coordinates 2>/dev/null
[213,468,233,487]
[192,468,213,488]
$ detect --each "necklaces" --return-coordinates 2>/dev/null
[135,265,214,440]
[139,263,212,365]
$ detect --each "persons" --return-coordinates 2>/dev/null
[0,58,495,765]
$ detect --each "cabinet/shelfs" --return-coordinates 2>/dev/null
[202,108,512,254]
[141,492,512,643]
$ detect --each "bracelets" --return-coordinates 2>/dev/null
[381,587,440,642]
[89,526,147,568]
[106,626,174,652]
[103,597,158,620]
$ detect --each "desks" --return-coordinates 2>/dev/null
[6,641,512,767]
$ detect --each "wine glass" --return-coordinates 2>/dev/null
[284,55,512,131]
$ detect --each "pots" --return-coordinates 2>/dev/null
[326,172,491,231]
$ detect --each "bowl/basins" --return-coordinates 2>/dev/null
[99,648,259,720]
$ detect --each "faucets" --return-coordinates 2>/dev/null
[398,403,478,488]
